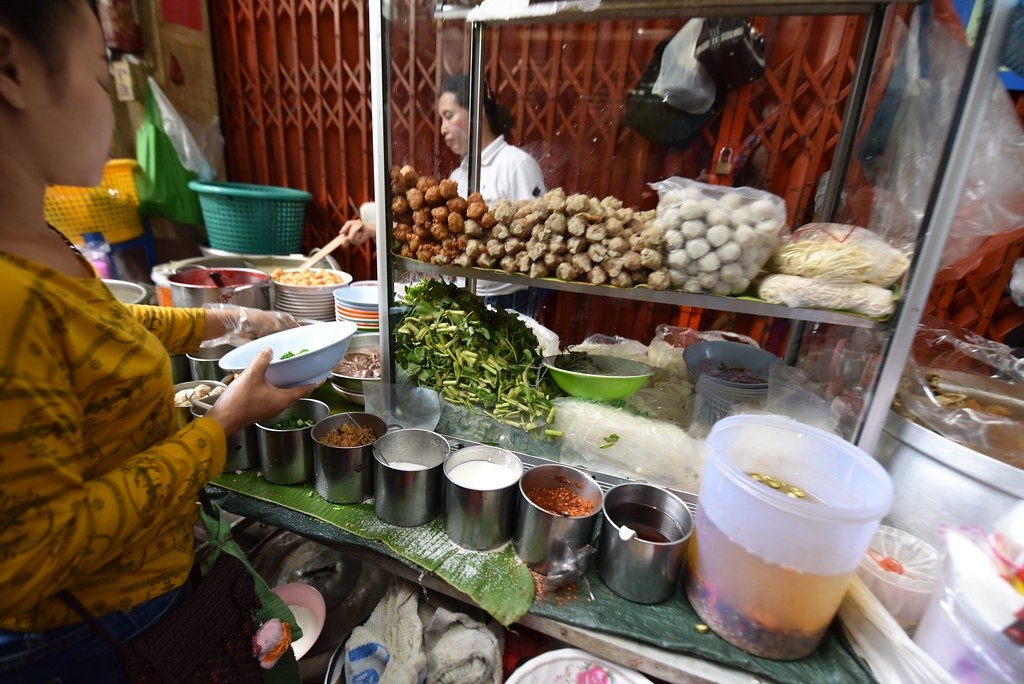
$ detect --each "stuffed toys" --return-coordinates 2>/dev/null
[251,618,293,670]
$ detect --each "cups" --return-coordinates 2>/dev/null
[311,412,405,505]
[185,347,233,380]
[191,392,260,472]
[173,380,227,429]
[254,398,331,486]
[599,482,694,607]
[443,444,525,550]
[371,427,464,527]
[512,464,605,578]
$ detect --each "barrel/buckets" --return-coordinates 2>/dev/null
[99,278,146,305]
[682,414,891,657]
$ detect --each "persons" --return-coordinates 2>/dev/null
[337,74,548,318]
[0,0,327,684]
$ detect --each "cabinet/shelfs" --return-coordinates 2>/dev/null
[366,0,1018,532]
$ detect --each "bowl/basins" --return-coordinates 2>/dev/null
[913,554,1024,684]
[270,583,327,661]
[859,523,937,636]
[875,366,1023,545]
[541,353,651,399]
[331,334,381,408]
[684,341,785,403]
[271,268,397,333]
[217,320,358,389]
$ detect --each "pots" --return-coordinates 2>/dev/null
[167,264,275,308]
[185,254,332,272]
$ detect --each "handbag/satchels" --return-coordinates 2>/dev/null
[133,84,202,226]
[694,13,765,92]
[652,17,715,114]
[624,35,724,149]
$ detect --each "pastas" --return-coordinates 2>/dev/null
[757,235,912,318]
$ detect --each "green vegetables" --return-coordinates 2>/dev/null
[391,275,619,449]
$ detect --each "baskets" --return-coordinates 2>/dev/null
[186,179,312,255]
[42,158,143,249]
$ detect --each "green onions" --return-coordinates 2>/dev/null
[270,412,314,430]
[280,348,308,360]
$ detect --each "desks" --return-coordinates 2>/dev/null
[193,475,881,684]
